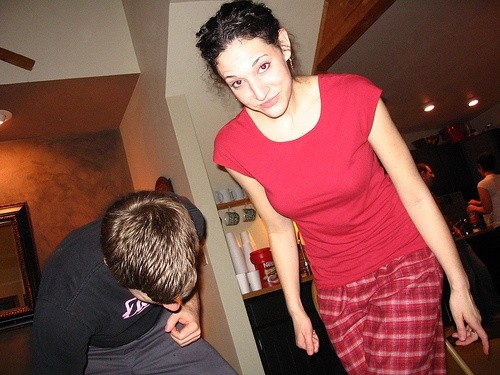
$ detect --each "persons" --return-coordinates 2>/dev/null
[32,190,238,375]
[196,0,489,375]
[416,162,434,187]
[466,152,500,241]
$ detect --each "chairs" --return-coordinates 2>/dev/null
[310,281,500,375]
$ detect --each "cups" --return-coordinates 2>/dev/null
[241,203,257,223]
[224,231,263,296]
[225,206,239,226]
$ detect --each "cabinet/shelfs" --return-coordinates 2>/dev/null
[245,281,348,375]
[411,127,500,202]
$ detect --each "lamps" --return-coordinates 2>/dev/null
[0,110,13,125]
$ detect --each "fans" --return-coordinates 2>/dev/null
[0,47,35,71]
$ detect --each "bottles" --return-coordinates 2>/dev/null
[250,247,280,290]
[469,211,480,228]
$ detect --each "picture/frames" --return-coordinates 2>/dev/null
[0,201,43,334]
